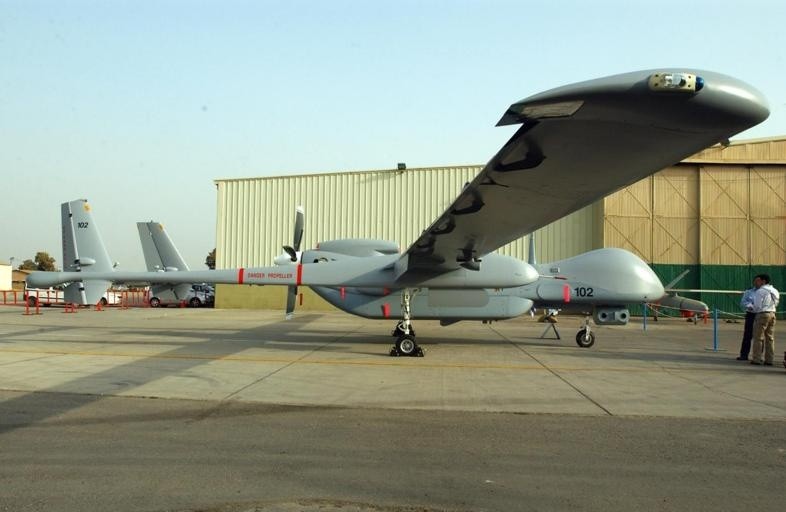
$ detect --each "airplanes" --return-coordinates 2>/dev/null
[644,264,713,322]
[23,68,771,358]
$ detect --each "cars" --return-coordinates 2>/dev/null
[143,283,215,308]
[26,273,122,308]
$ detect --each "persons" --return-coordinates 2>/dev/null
[736,274,764,363]
[750,274,780,365]
